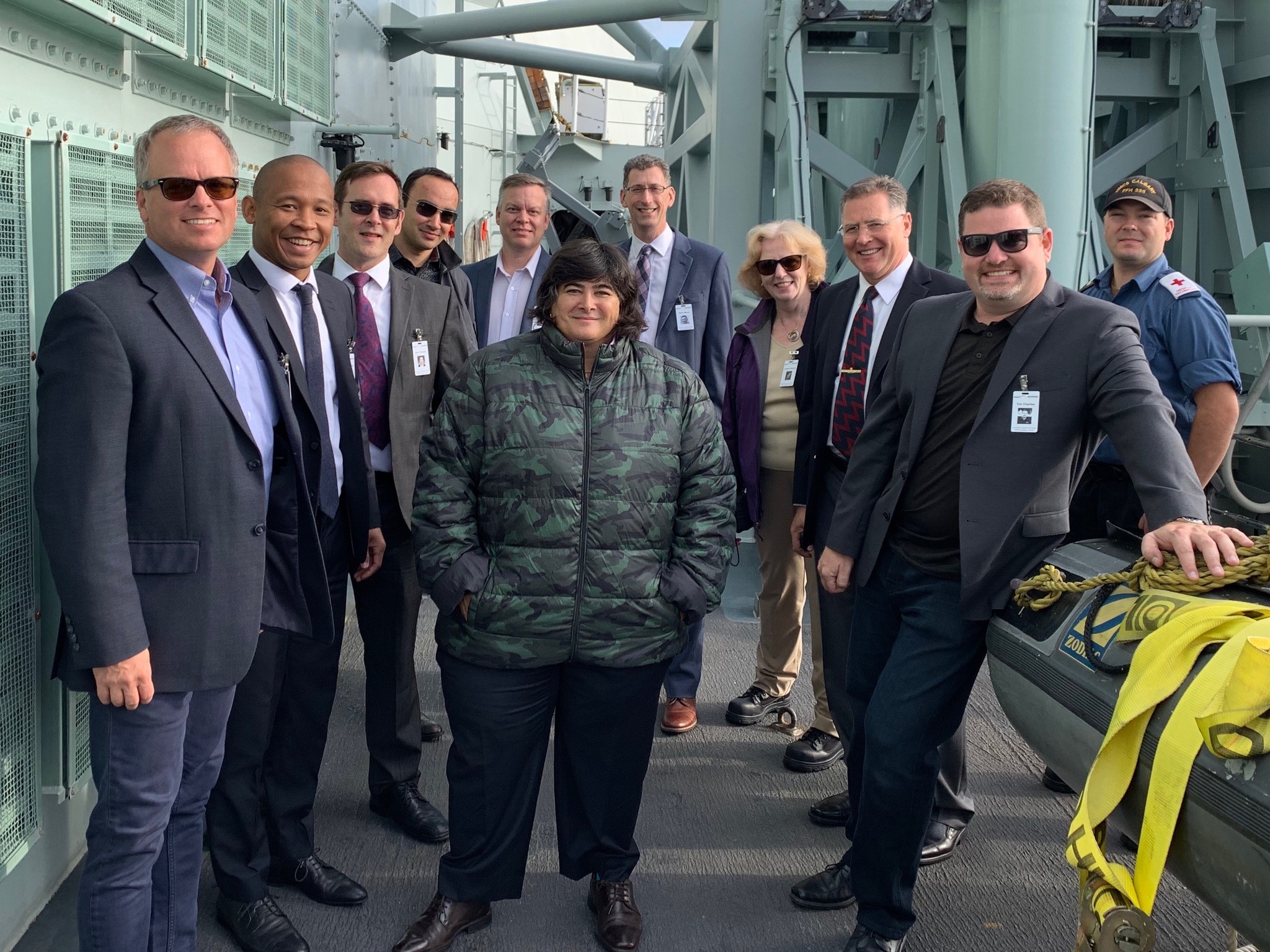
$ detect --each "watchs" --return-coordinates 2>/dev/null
[1172,516,1205,524]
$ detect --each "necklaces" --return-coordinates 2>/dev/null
[776,293,811,341]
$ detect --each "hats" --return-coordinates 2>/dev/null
[1102,175,1172,218]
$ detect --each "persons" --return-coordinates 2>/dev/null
[533,320,543,329]
[791,175,976,863]
[681,315,689,324]
[1042,173,1244,854]
[390,168,475,742]
[720,218,845,773]
[315,161,451,846]
[1017,409,1031,424]
[616,155,736,732]
[784,371,791,380]
[418,356,425,367]
[37,114,335,952]
[459,174,554,349]
[789,180,1254,952]
[391,239,735,952]
[205,155,387,952]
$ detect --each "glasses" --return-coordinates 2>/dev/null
[138,177,238,201]
[960,226,1043,257]
[625,185,673,195]
[754,254,802,276]
[337,200,402,219]
[837,214,905,238]
[402,192,459,224]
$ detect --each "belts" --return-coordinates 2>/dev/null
[829,450,849,474]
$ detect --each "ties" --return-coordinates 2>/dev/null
[292,283,340,521]
[349,272,390,451]
[831,286,880,461]
[634,245,657,341]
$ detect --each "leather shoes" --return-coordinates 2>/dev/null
[214,889,311,952]
[919,820,967,864]
[726,684,791,725]
[586,874,645,951]
[809,788,850,827]
[660,695,697,734]
[268,847,368,906]
[789,858,855,910]
[390,892,493,952]
[844,922,908,952]
[783,726,843,771]
[368,781,451,842]
